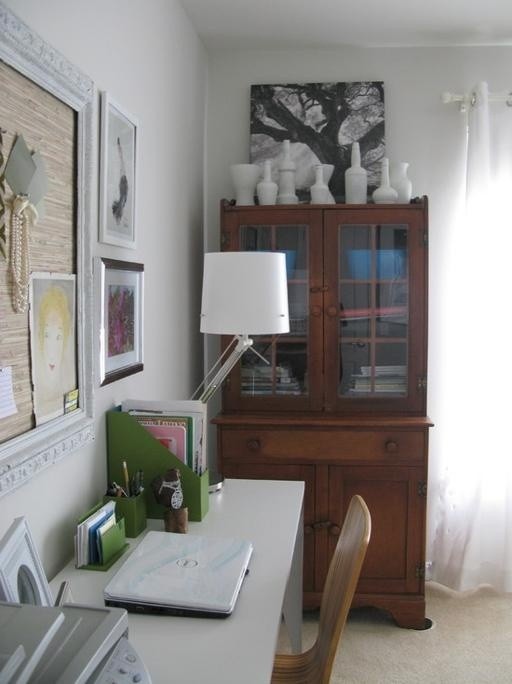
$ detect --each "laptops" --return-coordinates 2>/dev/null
[103,531,254,619]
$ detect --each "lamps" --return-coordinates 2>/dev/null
[185,243,292,413]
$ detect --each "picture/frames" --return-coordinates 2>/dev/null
[98,257,146,386]
[0,515,62,608]
[1,1,100,493]
[97,86,145,250]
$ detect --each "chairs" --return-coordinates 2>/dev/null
[270,490,374,682]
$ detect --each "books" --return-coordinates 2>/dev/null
[117,398,208,477]
[238,363,302,395]
[349,363,408,397]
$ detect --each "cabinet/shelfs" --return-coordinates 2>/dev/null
[215,197,430,414]
[211,414,435,631]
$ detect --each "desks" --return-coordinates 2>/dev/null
[46,470,312,682]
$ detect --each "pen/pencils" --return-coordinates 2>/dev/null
[113,460,145,498]
